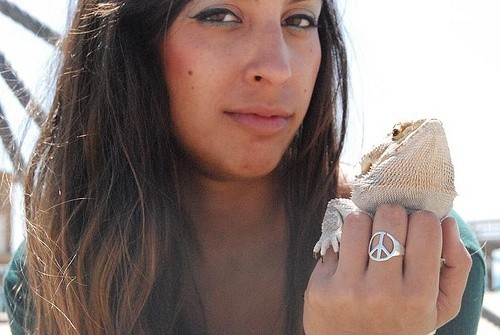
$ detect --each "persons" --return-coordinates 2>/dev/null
[4,0,489,334]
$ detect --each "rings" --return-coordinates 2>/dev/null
[367,231,406,264]
